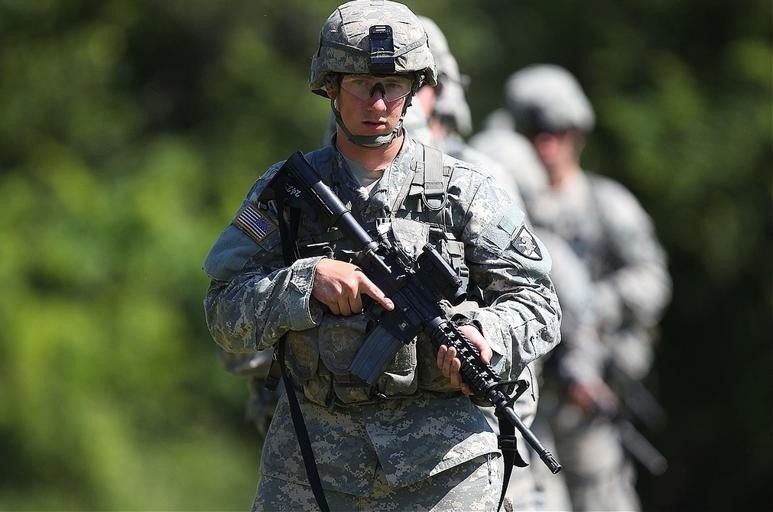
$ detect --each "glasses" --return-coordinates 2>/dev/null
[334,73,417,102]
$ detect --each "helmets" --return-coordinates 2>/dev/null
[505,64,594,134]
[308,1,473,137]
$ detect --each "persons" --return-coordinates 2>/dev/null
[406,15,672,512]
[203,0,562,512]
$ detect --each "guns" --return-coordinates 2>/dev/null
[256,151,561,474]
